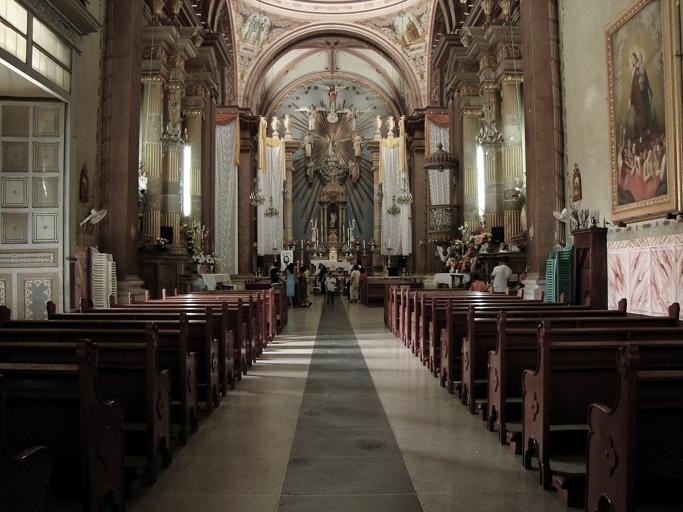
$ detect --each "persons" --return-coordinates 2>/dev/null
[616,50,666,200]
[138,167,149,204]
[270,105,395,184]
[515,176,527,234]
[190,272,209,291]
[256,261,368,309]
[446,256,526,296]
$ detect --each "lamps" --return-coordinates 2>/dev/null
[376,0,413,215]
[475,0,506,159]
[327,83,338,123]
[160,0,188,144]
[264,0,280,218]
[250,0,265,207]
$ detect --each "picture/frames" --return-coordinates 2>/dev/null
[571,162,582,202]
[80,162,89,203]
[604,0,683,225]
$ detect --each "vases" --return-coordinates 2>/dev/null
[196,264,201,273]
[210,265,213,274]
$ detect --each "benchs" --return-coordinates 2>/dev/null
[0,286,287,512]
[385,285,683,512]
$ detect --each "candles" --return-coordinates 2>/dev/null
[274,240,391,249]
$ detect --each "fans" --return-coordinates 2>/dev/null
[80,208,107,224]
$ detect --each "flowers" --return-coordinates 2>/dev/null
[192,251,206,264]
[206,252,220,264]
